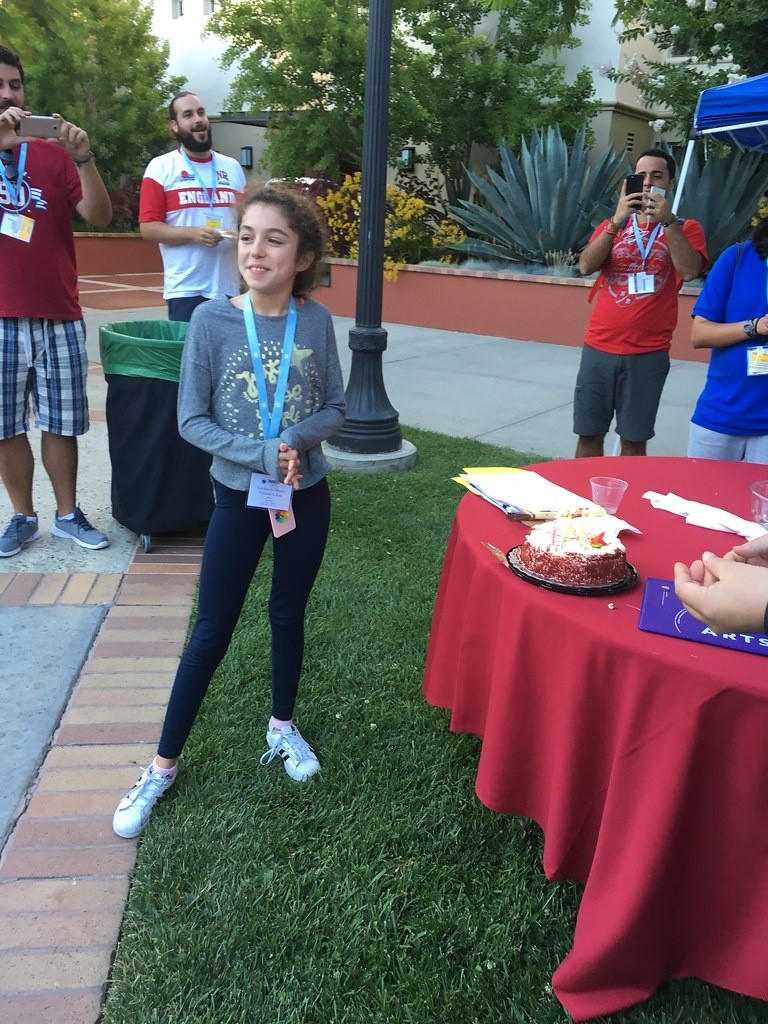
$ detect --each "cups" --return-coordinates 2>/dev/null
[750,480,768,561]
[590,477,628,514]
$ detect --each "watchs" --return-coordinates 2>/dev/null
[742,316,757,339]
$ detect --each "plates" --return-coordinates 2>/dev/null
[505,545,639,596]
[216,231,239,239]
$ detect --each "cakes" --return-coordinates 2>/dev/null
[519,516,626,586]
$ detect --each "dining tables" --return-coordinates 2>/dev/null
[422,454,768,1022]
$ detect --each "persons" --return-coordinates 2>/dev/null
[0,44,112,558]
[112,186,349,840]
[573,149,710,459]
[674,532,768,639]
[687,188,768,466]
[138,92,246,324]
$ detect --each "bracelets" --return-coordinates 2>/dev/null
[753,318,764,337]
[70,150,95,167]
[602,219,621,236]
[662,213,675,227]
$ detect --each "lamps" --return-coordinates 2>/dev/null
[401,146,416,170]
[240,144,252,168]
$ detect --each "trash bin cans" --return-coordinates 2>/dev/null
[97,318,214,555]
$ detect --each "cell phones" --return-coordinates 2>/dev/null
[626,174,644,210]
[20,116,61,138]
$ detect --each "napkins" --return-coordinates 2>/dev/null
[642,489,767,541]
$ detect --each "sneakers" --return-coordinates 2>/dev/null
[0,512,39,557]
[260,723,321,782]
[113,764,178,838]
[51,502,110,549]
